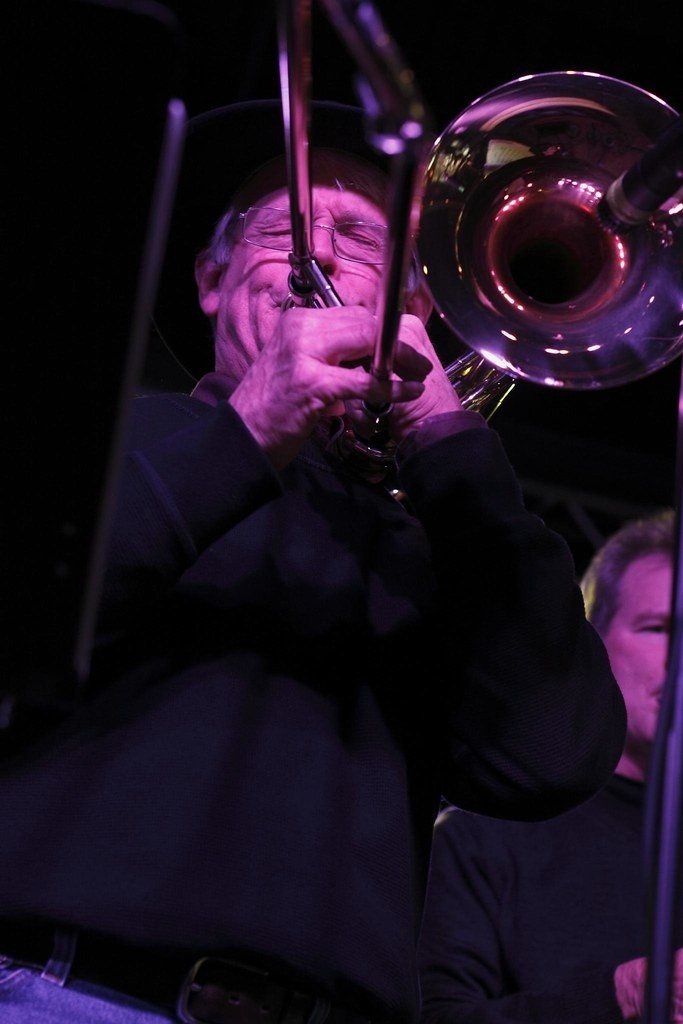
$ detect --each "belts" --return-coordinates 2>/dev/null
[0,916,372,1024]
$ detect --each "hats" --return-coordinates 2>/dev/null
[166,100,436,240]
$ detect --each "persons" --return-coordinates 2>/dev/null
[421,509,682,1024]
[1,151,627,1024]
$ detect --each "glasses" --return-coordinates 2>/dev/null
[238,207,419,286]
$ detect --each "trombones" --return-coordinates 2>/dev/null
[275,0,683,496]
